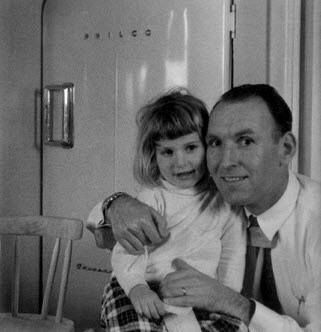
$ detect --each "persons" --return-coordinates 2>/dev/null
[99,92,248,332]
[85,84,321,332]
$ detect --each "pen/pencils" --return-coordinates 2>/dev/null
[296,295,305,315]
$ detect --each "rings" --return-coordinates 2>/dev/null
[181,287,187,296]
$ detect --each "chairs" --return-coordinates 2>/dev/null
[0,215,84,332]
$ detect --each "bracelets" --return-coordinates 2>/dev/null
[100,192,127,221]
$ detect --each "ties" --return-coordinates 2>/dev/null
[248,215,284,313]
